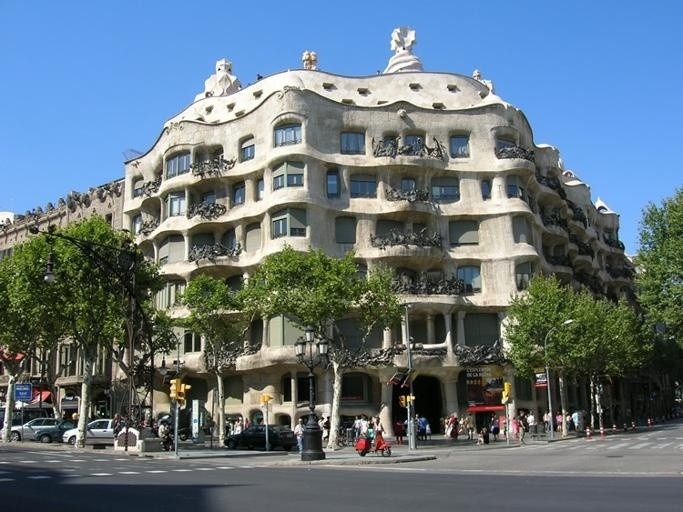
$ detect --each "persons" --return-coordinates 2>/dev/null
[111,413,175,452]
[206,417,263,438]
[72,410,79,427]
[293,408,589,455]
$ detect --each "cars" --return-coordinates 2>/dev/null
[61,419,127,445]
[8,418,71,443]
[224,425,299,451]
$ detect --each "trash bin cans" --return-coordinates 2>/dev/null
[346,428,354,447]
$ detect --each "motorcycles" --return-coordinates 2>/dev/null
[353,422,392,458]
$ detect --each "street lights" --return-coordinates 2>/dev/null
[162,328,197,456]
[404,301,418,450]
[543,317,574,440]
[297,324,330,461]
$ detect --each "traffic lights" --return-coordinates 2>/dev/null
[501,382,514,407]
[170,378,192,410]
[260,394,270,414]
[399,394,415,407]
